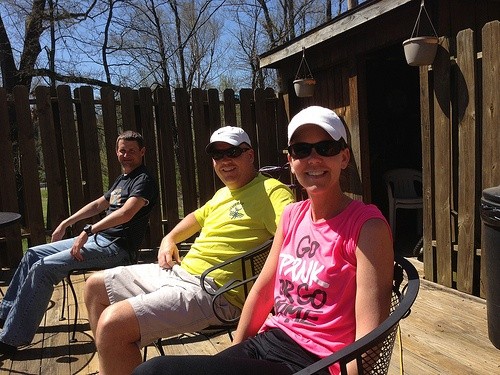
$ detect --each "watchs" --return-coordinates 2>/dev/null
[83,224,93,237]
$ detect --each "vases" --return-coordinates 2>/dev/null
[402,37,439,66]
[293,79,316,97]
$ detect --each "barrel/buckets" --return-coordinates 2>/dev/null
[293,78,316,97]
[401,37,438,66]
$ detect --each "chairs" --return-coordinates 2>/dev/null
[143,237,274,361]
[382,168,422,241]
[59,205,153,343]
[212,251,420,375]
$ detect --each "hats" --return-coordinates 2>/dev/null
[287,106,348,147]
[205,125,252,155]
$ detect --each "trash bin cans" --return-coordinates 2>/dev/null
[481,185,500,350]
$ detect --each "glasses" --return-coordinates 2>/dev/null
[209,146,250,161]
[287,140,345,159]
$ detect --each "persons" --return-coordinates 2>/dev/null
[132,105,394,375]
[0,130,158,358]
[83,125,298,375]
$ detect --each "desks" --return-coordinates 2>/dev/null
[0,212,23,286]
